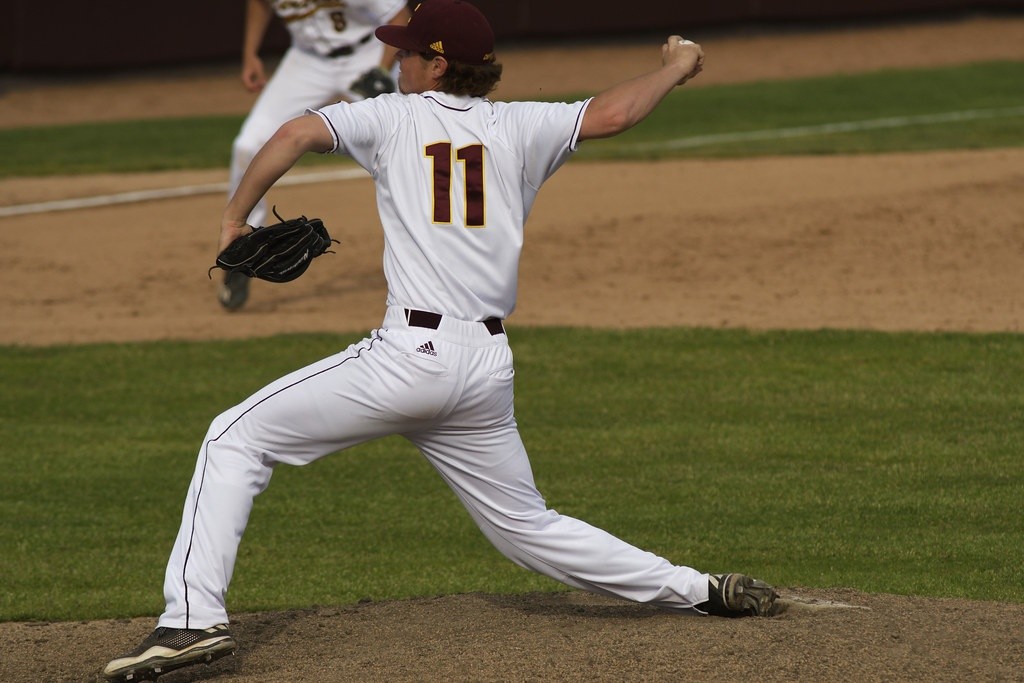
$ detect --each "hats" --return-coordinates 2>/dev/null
[375,0,497,65]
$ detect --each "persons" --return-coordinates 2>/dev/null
[219,0,411,311]
[103,0,780,683]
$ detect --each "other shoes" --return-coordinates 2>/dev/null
[218,272,250,312]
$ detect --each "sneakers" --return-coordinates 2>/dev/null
[693,573,790,617]
[103,623,237,683]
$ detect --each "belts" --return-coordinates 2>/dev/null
[405,309,504,336]
[327,34,372,58]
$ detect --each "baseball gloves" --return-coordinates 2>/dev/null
[215,214,332,284]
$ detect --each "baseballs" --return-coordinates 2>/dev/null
[677,38,697,46]
